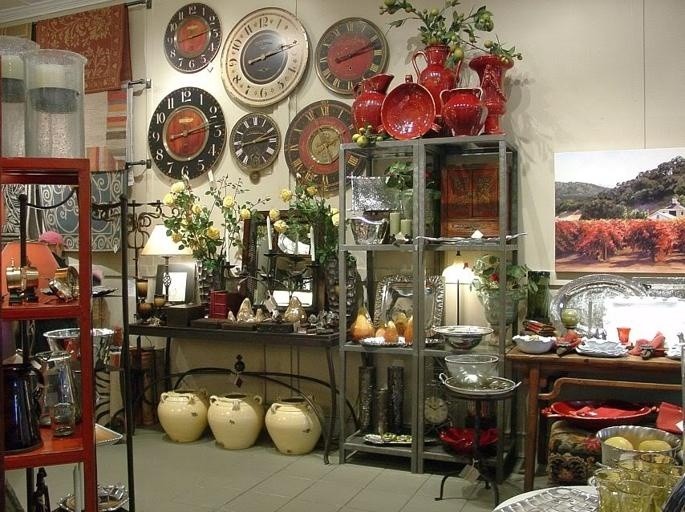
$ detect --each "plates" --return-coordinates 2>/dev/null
[358,334,442,348]
[551,273,653,359]
[433,325,495,335]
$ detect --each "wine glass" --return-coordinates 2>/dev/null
[478,289,526,348]
[97,481,123,508]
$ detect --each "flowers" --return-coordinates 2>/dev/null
[161,171,273,274]
[267,171,356,264]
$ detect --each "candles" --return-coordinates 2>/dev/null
[390,213,400,236]
[401,219,412,237]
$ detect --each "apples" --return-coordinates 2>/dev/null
[604,436,633,451]
[639,440,671,451]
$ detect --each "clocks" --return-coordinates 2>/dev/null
[218,7,310,109]
[162,2,222,74]
[283,100,365,191]
[314,17,387,96]
[423,373,453,437]
[147,86,226,184]
[229,112,282,172]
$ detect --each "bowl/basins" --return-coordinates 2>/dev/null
[513,334,556,354]
[344,216,390,245]
[42,329,114,370]
[595,425,681,468]
[381,75,444,140]
[445,354,501,386]
[543,398,658,429]
[445,336,482,350]
[439,429,499,454]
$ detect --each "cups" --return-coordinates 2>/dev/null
[596,451,685,510]
[616,327,631,343]
[24,50,88,160]
[0,33,40,158]
[50,402,75,435]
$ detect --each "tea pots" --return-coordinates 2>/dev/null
[412,43,462,128]
[0,349,43,454]
[353,74,395,139]
[36,351,82,428]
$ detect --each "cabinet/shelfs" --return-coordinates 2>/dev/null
[417,134,520,476]
[0,154,100,511]
[337,139,417,474]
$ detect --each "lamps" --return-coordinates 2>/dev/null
[440,250,478,325]
[140,225,195,309]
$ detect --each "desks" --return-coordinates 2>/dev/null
[128,320,358,466]
[505,339,685,494]
[492,483,605,512]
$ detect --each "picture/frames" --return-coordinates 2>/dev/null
[242,209,324,313]
[372,273,445,337]
[155,264,194,304]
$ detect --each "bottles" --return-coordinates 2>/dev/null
[526,270,552,333]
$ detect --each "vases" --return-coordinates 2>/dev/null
[350,74,393,137]
[469,56,516,134]
[410,46,462,117]
[324,260,361,322]
[195,259,226,317]
